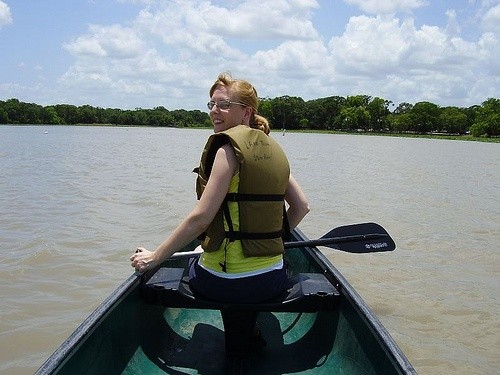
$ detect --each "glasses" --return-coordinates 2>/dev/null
[207,101,248,109]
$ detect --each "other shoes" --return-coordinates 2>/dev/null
[223,331,266,372]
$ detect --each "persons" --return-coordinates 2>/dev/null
[129,71,311,352]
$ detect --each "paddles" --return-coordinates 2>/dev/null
[137,220,396,275]
